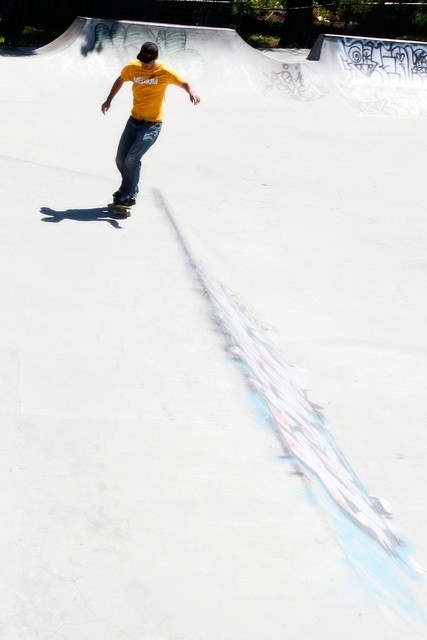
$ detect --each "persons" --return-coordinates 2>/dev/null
[100,42,200,207]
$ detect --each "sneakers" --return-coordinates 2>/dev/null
[113,186,138,198]
[113,194,136,206]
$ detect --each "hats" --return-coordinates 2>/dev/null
[137,42,158,63]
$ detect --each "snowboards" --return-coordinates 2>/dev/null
[113,198,131,219]
